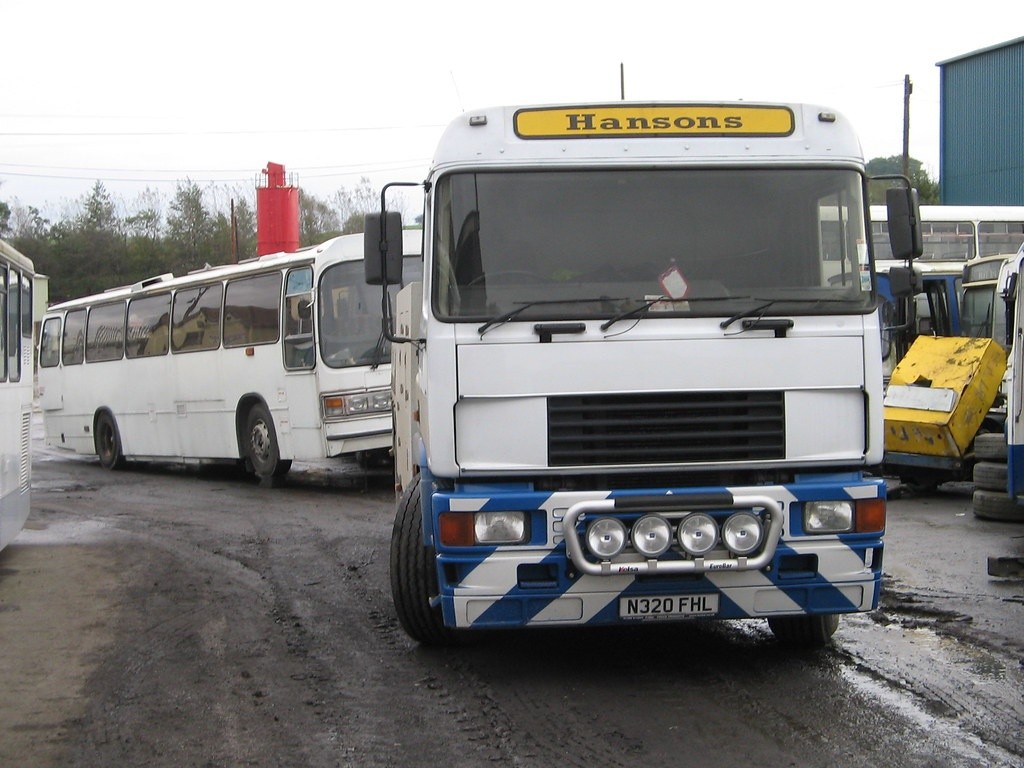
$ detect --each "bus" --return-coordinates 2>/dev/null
[37,229,426,482]
[817,203,1024,500]
[0,242,32,560]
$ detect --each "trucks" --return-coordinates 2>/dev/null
[363,96,926,648]
